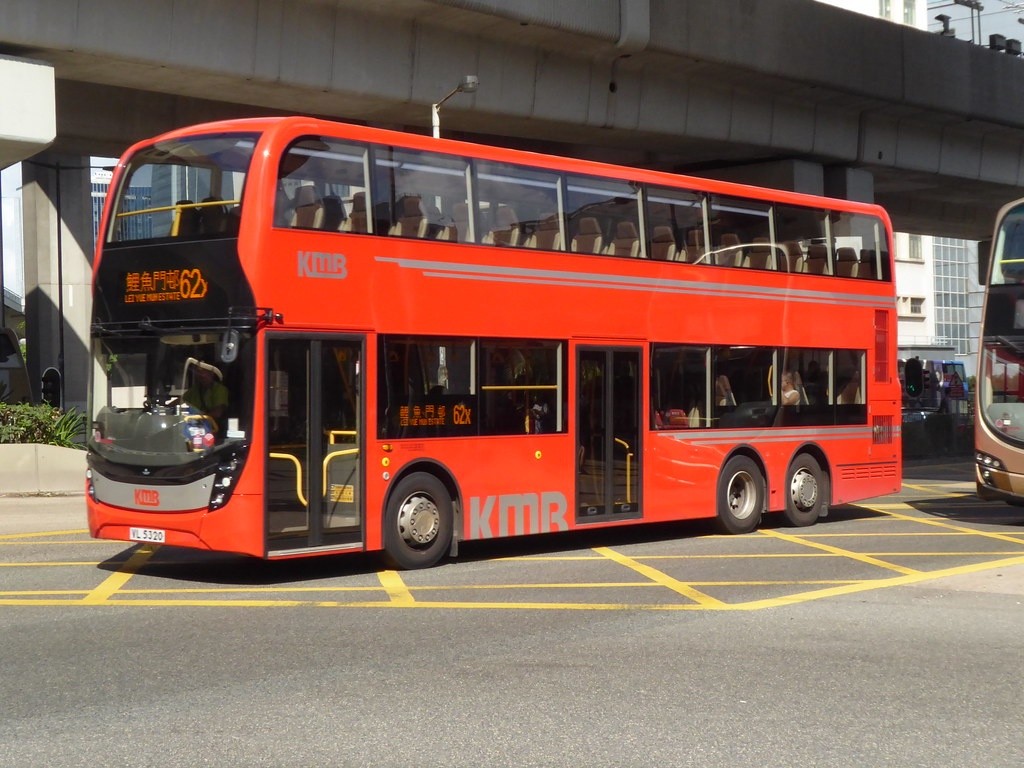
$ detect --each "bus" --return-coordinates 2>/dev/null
[897,357,969,416]
[83,117,900,569]
[0,328,34,407]
[975,196,1024,509]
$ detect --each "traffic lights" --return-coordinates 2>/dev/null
[41,367,63,411]
[904,358,924,399]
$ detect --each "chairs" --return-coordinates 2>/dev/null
[737,358,861,404]
[169,179,878,279]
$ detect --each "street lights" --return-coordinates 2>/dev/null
[430,74,479,393]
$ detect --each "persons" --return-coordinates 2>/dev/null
[781,371,801,406]
[165,362,229,421]
